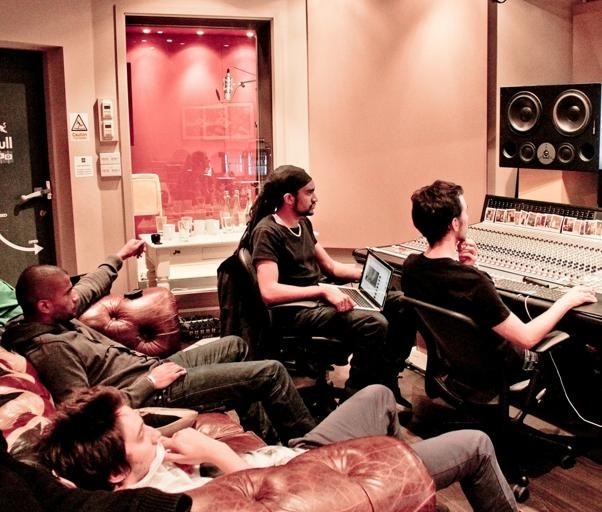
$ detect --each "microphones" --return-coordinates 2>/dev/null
[224,67,232,100]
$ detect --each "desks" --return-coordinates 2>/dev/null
[353,249,602,364]
[138,233,244,288]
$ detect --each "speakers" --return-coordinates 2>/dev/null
[499,83,601,171]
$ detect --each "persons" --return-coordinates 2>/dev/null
[399,181,598,388]
[36,384,518,512]
[244,164,404,391]
[4,238,316,448]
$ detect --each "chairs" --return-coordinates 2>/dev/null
[396,295,577,501]
[234,245,346,425]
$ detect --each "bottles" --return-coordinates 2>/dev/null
[219,189,253,233]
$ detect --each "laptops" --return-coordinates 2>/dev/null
[317,249,394,312]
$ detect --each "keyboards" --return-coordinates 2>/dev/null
[494,278,566,302]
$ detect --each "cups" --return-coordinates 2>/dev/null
[150,215,220,245]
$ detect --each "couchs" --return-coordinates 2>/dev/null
[0,287,435,512]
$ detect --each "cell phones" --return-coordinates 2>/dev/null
[124,289,142,299]
